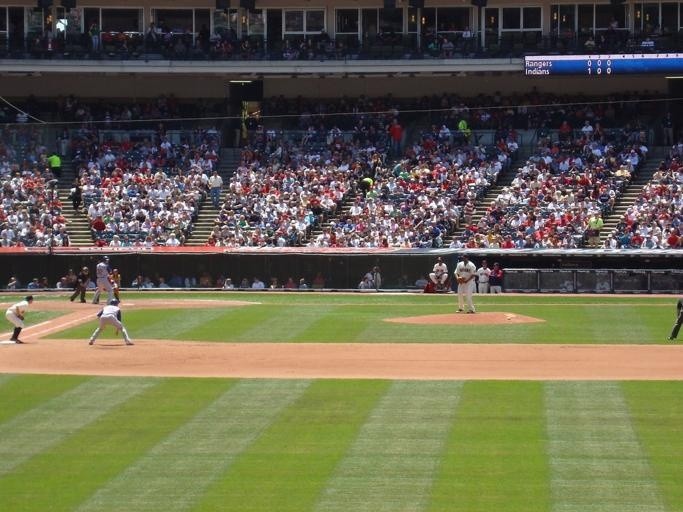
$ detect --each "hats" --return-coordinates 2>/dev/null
[23,295,33,301]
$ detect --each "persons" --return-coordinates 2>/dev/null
[87,297,136,346]
[90,255,113,305]
[667,297,683,341]
[0,12,683,294]
[4,294,33,343]
[96,268,121,304]
[453,253,478,314]
[68,266,90,303]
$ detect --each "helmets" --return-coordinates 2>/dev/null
[109,298,118,306]
